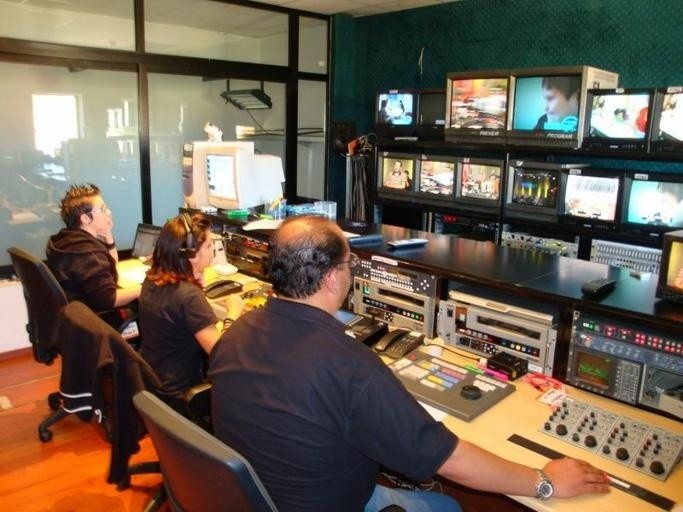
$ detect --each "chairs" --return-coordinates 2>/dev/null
[6,243,128,442]
[127,383,404,511]
[55,300,181,486]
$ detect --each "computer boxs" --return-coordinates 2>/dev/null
[181,141,255,211]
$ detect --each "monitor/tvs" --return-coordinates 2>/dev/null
[373,64,683,238]
[196,141,285,215]
[130,223,161,260]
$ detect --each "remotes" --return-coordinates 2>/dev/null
[581,278,617,296]
[348,233,383,245]
[386,239,429,249]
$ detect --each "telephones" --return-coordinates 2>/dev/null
[202,279,243,298]
[373,328,425,359]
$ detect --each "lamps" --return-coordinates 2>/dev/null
[220,76,269,115]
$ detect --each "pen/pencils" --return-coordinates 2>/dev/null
[477,365,509,380]
[604,475,631,490]
[270,194,283,210]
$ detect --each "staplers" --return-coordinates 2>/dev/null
[248,211,274,221]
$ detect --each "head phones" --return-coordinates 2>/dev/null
[175,212,197,260]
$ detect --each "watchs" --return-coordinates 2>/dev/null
[535,469,553,502]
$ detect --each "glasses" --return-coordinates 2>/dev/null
[341,253,361,268]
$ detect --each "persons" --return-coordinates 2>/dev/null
[387,161,409,190]
[404,171,413,191]
[533,76,581,132]
[137,213,246,402]
[46,183,143,329]
[208,213,611,512]
